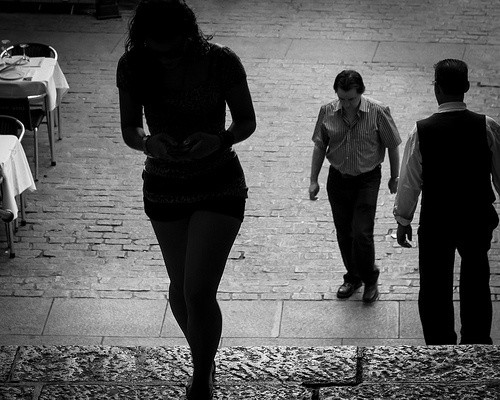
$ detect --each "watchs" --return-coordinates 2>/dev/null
[143,135,156,159]
[391,176,399,182]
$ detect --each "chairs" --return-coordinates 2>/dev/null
[0,44,63,258]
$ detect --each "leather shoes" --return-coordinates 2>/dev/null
[337,282,362,299]
[362,285,379,303]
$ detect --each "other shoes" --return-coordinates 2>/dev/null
[186,361,217,400]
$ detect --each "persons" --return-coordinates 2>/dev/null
[309,70,403,305]
[116,0,258,400]
[393,59,500,345]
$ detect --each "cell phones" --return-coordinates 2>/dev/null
[166,135,193,155]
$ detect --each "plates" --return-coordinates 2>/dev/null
[5,55,29,65]
[0,70,23,79]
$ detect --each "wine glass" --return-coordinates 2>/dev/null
[20,43,30,63]
[2,39,11,59]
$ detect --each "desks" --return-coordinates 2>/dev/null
[0,136,36,231]
[0,56,70,167]
